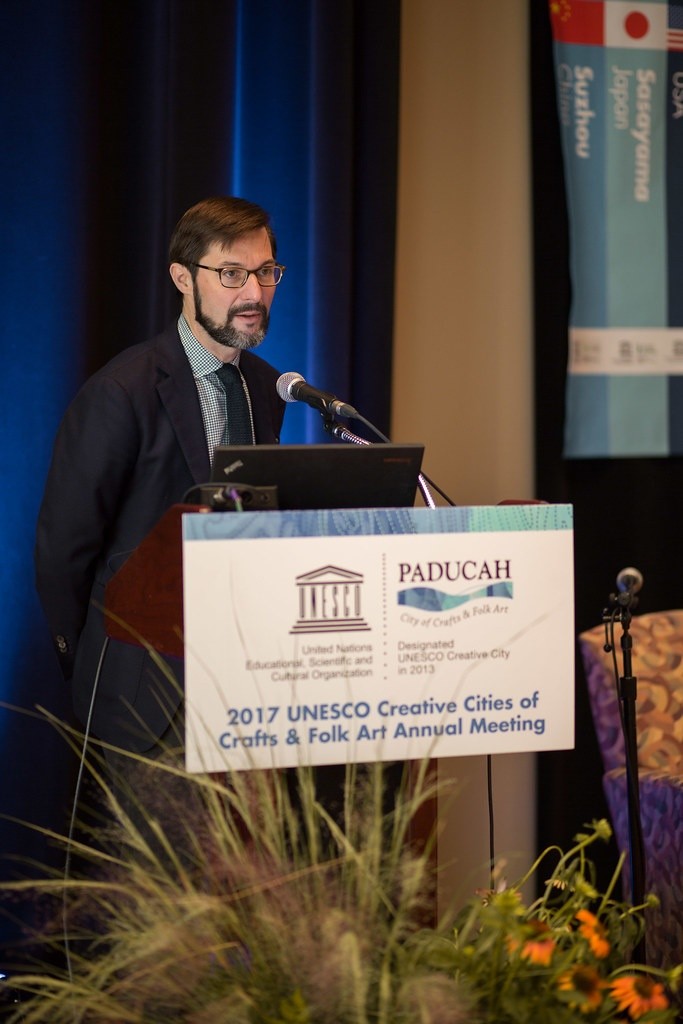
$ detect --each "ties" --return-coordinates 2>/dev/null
[215,363,254,445]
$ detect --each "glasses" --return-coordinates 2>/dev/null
[196,264,286,288]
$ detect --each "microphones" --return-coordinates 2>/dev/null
[616,566,643,595]
[276,371,358,419]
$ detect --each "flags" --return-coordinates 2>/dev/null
[549,0,683,454]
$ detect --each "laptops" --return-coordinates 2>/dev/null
[201,443,426,514]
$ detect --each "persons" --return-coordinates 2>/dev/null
[33,190,296,938]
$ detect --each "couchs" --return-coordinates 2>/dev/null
[581,610,683,1024]
